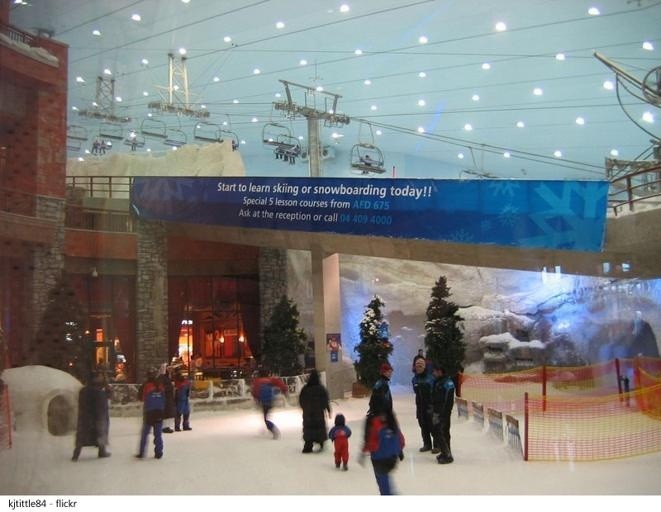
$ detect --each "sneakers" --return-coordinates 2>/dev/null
[420,446,453,464]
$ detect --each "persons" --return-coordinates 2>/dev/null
[298,370,332,454]
[91,140,99,153]
[99,140,107,155]
[137,363,192,460]
[328,414,352,471]
[361,389,405,495]
[412,348,455,464]
[275,142,300,164]
[362,155,371,175]
[73,372,112,462]
[365,363,394,415]
[251,367,290,440]
